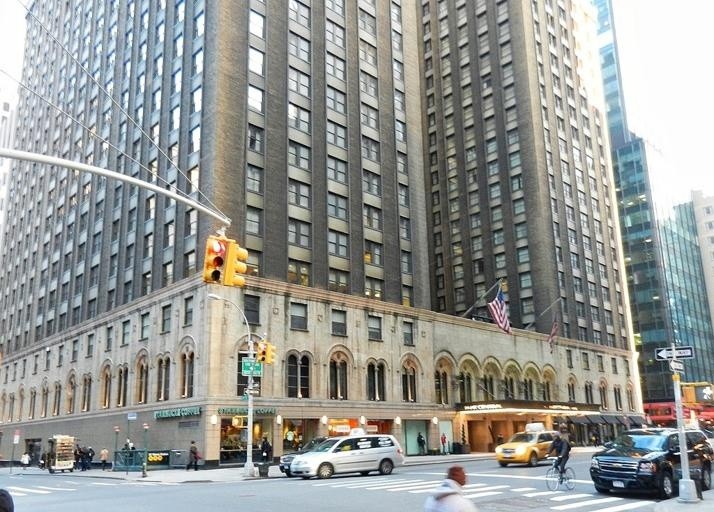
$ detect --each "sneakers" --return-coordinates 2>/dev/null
[557,471,566,484]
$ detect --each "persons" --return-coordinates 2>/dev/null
[100,446,109,471]
[441,432,447,453]
[592,429,600,447]
[695,435,713,490]
[424,465,480,512]
[544,431,571,474]
[417,432,425,456]
[261,436,271,462]
[0,489,14,512]
[186,441,199,471]
[20,452,32,470]
[73,443,95,472]
[122,443,141,466]
[39,450,47,469]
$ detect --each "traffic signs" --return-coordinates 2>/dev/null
[241,356,262,378]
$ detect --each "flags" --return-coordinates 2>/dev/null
[549,320,559,355]
[487,283,513,334]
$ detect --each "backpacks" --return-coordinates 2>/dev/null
[560,438,571,452]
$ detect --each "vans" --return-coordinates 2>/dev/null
[592,428,714,498]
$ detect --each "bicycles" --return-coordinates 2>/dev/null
[542,454,576,492]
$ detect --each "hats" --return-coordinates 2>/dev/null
[448,466,466,485]
[551,431,560,438]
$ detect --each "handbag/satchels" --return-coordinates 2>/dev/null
[195,453,202,460]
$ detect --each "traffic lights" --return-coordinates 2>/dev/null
[205,240,227,283]
[260,343,268,362]
[235,247,249,289]
[272,346,276,363]
[695,383,713,403]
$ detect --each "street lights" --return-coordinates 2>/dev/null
[207,291,255,477]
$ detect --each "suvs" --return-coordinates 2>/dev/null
[278,436,332,478]
[494,428,560,467]
[289,435,404,480]
